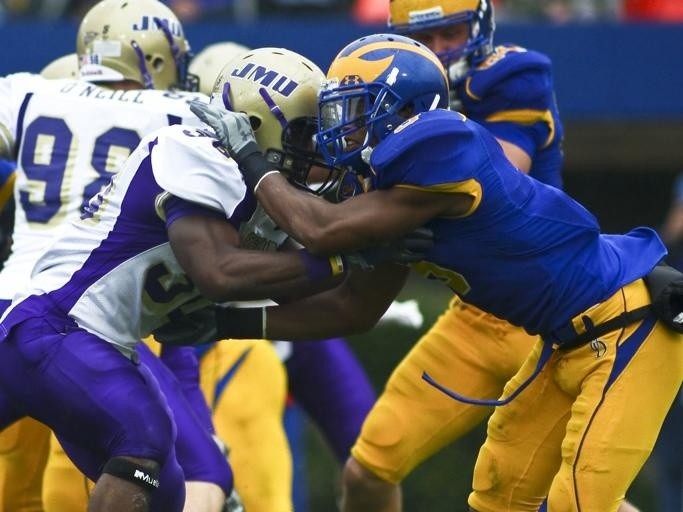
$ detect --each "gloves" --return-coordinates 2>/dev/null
[150,303,267,346]
[339,224,436,272]
[185,99,263,173]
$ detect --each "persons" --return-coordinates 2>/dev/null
[0,0,682,511]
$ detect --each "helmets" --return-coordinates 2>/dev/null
[186,41,251,98]
[76,0,199,93]
[389,0,496,90]
[210,46,344,196]
[315,33,451,168]
[39,52,78,80]
